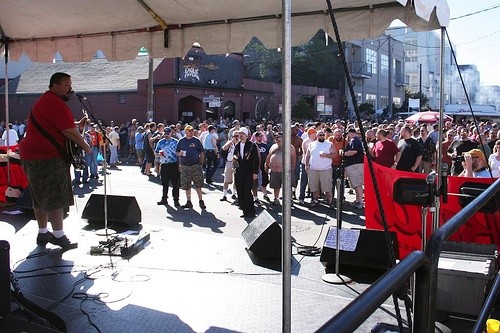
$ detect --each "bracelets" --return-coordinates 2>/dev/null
[394,162,397,165]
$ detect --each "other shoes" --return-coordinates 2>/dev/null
[199,200,206,209]
[181,201,193,208]
[157,200,168,205]
[174,201,180,207]
[47,233,78,249]
[232,194,236,199]
[111,160,122,167]
[35,231,51,246]
[350,200,363,209]
[255,193,336,209]
[72,178,89,185]
[220,196,226,201]
[239,212,255,218]
[141,169,154,176]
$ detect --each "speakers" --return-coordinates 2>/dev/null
[241,210,282,254]
[319,226,398,275]
[80,194,141,227]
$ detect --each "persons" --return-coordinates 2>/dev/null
[0,119,27,146]
[305,130,338,209]
[175,125,206,209]
[20,72,92,250]
[266,132,296,206]
[154,127,180,207]
[233,127,259,219]
[72,118,500,208]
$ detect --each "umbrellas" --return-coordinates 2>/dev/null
[406,111,452,122]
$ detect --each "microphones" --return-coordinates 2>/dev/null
[342,95,349,107]
[71,91,88,101]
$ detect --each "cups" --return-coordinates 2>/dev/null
[157,134,160,138]
[319,151,324,156]
[159,150,164,156]
[486,318,500,333]
[339,150,343,155]
[182,151,186,156]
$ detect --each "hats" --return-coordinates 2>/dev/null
[238,126,248,137]
[184,125,194,130]
[447,129,457,136]
[308,128,316,134]
[461,148,485,161]
[274,132,282,137]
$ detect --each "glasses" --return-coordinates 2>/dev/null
[163,132,170,136]
[318,134,325,136]
